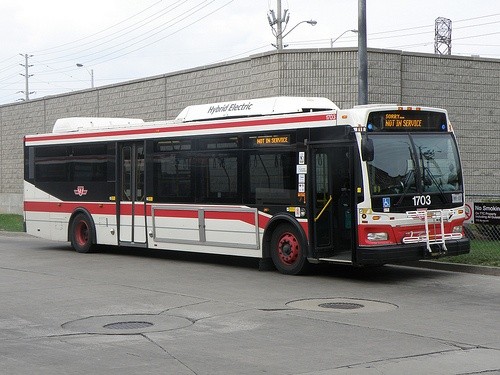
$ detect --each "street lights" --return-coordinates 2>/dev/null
[76,63,94,88]
[330,30,358,48]
[282,20,317,39]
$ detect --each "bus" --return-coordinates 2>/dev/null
[22,96,471,275]
